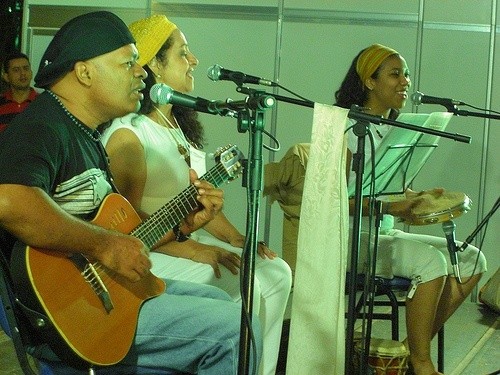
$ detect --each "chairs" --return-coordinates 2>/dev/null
[0,251,180,375]
[345,272,445,375]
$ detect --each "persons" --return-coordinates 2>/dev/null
[335,43,488,375]
[0,11,264,375]
[0,53,42,131]
[101,13,292,375]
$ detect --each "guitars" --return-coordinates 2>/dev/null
[9,142,247,371]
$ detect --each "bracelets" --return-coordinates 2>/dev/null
[385,202,391,215]
[173,224,190,242]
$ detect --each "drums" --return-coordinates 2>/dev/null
[400,191,471,226]
[344,332,407,375]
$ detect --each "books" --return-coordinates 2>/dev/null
[349,110,454,197]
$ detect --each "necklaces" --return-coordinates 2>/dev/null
[47,89,102,141]
[153,106,190,167]
[372,122,384,138]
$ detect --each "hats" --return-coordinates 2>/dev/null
[128,15,177,67]
[33,11,136,89]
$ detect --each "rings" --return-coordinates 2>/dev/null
[260,241,266,245]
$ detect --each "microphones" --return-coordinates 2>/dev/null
[150,83,239,119]
[410,91,464,105]
[207,64,276,87]
[442,219,461,283]
